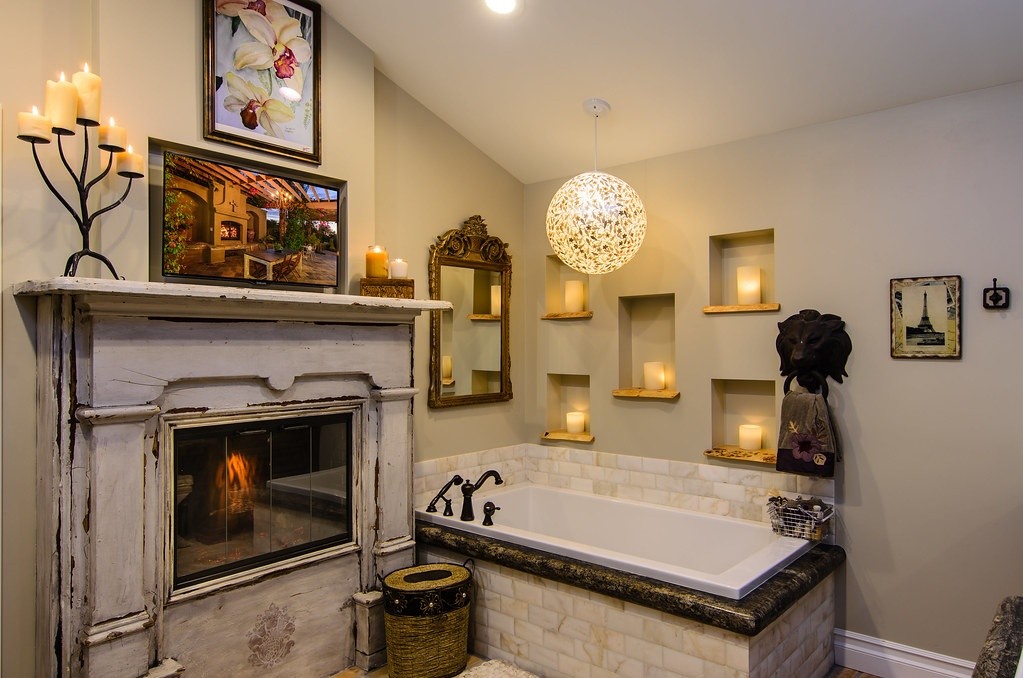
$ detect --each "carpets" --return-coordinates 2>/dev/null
[455,658,539,678]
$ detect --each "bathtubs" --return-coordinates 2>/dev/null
[414,482,835,678]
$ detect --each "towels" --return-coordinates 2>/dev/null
[775,389,842,478]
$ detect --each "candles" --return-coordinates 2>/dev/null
[491,285,501,316]
[443,356,452,379]
[98,116,128,153]
[116,145,145,179]
[738,425,762,450]
[737,266,761,305]
[17,106,52,145]
[365,245,388,278]
[567,412,585,433]
[44,71,78,136]
[71,62,102,126]
[565,280,583,312]
[644,361,664,389]
[390,258,408,278]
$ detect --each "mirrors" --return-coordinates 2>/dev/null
[427,215,514,408]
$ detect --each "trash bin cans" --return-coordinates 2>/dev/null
[376,558,475,678]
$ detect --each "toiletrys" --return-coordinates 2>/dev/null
[766,496,828,541]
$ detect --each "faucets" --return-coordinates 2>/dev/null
[460,469,504,521]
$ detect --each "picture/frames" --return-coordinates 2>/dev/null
[889,275,962,359]
[147,137,350,295]
[202,0,323,164]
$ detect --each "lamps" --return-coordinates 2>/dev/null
[546,100,647,273]
